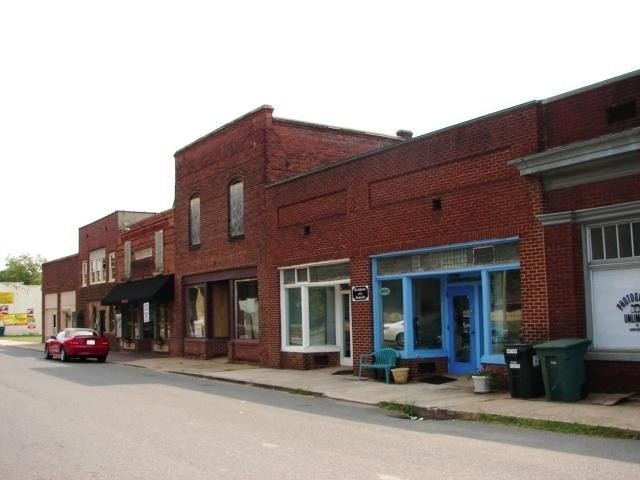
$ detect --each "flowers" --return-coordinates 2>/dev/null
[466,368,497,386]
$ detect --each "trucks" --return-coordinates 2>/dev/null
[459,302,521,342]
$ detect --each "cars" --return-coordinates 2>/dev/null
[417,302,441,343]
[383,319,404,347]
[44,327,110,363]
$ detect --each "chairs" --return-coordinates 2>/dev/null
[358,348,400,384]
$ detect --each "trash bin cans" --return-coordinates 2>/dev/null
[502,341,545,398]
[533,338,592,402]
[0,326,5,337]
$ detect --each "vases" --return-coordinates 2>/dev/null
[471,376,491,393]
[391,368,410,383]
